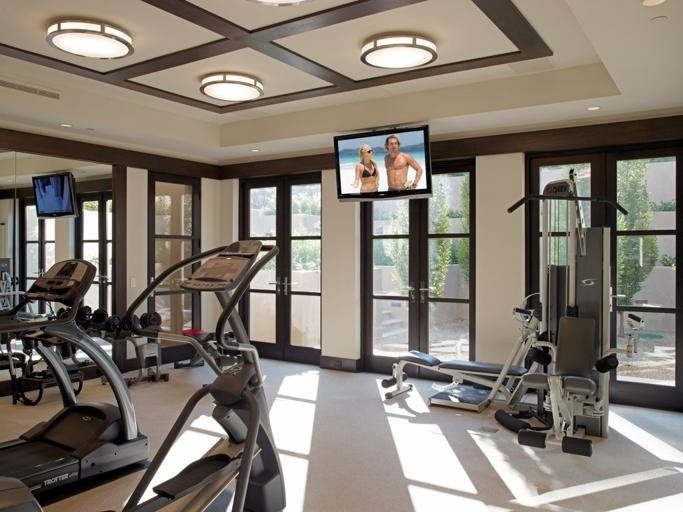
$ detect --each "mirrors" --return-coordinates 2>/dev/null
[0,130,127,397]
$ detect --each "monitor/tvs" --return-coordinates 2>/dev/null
[334,124,433,202]
[32,172,79,219]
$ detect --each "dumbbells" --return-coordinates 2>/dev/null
[152,374,170,381]
[56,306,161,331]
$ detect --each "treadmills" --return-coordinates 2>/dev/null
[0,259,149,506]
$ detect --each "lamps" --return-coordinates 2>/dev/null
[253,0,310,7]
[360,30,439,71]
[44,17,135,60]
[200,71,264,103]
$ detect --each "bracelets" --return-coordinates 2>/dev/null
[411,182,417,188]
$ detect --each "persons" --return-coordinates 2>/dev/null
[346,142,379,194]
[382,134,423,192]
